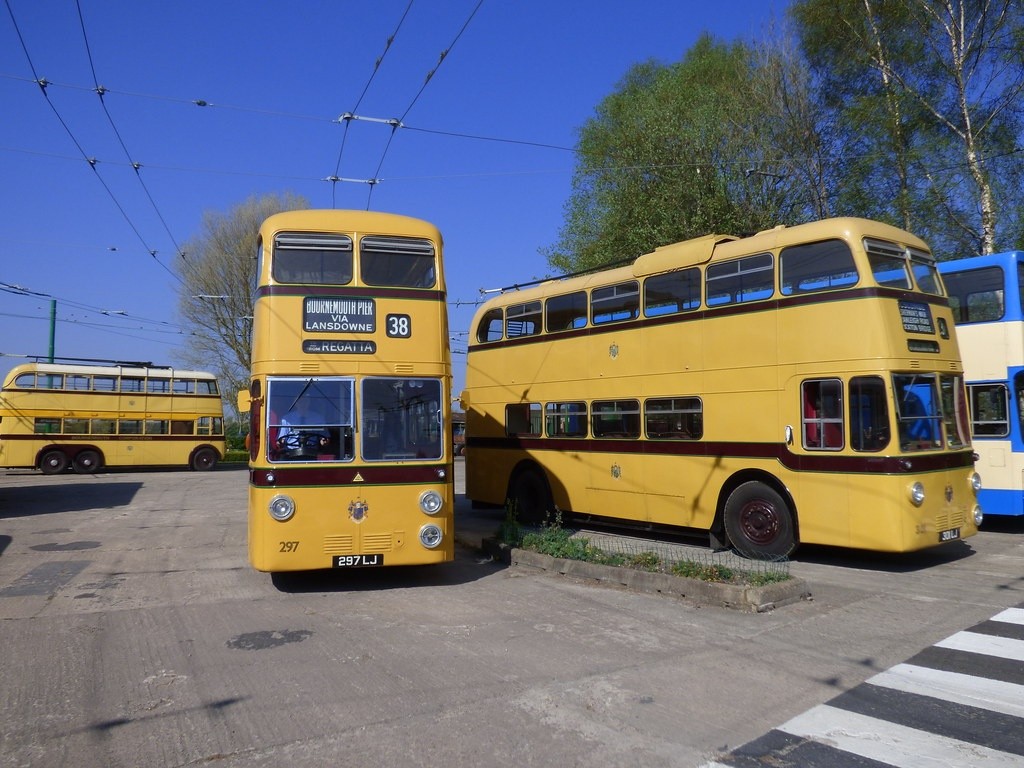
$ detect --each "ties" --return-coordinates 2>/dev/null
[300,415,306,435]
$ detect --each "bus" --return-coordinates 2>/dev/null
[1,362,227,475]
[466,215,985,560]
[236,210,471,573]
[572,251,1023,518]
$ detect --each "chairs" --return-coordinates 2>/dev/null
[549,429,700,440]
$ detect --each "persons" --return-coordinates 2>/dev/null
[276,387,331,450]
